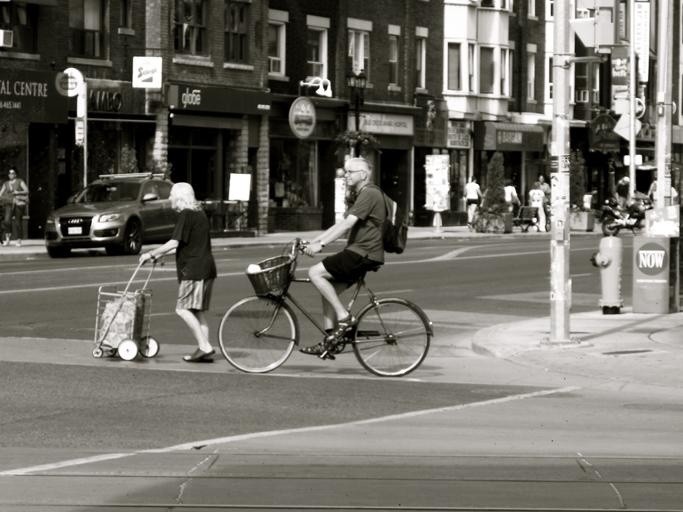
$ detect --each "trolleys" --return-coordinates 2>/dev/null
[91,251,159,363]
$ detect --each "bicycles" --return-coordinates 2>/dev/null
[217,237,433,379]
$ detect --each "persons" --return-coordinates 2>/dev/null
[613,176,679,208]
[528,174,551,233]
[299,155,387,354]
[0,167,30,247]
[503,178,522,214]
[462,174,483,234]
[136,178,216,362]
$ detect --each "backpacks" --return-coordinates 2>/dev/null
[382,191,410,254]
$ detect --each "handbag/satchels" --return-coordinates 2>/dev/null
[15,194,30,206]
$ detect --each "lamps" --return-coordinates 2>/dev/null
[299,75,334,98]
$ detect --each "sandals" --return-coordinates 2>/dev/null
[300,342,339,355]
[325,311,359,343]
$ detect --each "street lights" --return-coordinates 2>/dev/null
[344,68,368,156]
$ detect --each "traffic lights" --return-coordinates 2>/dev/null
[565,14,613,49]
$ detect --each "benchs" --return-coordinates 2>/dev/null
[508,205,541,234]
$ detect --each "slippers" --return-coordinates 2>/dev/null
[182,346,215,362]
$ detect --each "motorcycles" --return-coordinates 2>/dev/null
[599,191,654,237]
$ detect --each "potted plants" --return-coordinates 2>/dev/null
[567,147,595,233]
[470,149,513,235]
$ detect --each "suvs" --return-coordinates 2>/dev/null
[45,172,178,259]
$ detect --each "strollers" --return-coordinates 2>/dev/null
[0,190,18,244]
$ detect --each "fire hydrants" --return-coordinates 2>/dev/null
[589,236,623,316]
